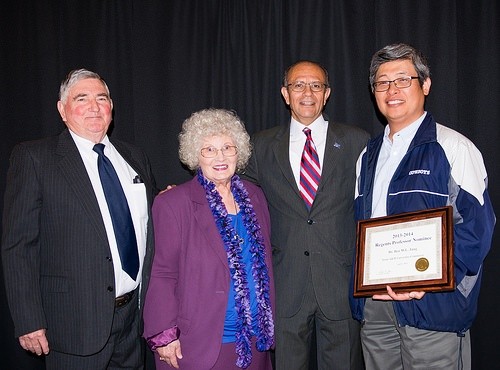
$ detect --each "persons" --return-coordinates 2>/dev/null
[143,107,275,370]
[158,60,372,370]
[2,69,178,370]
[350,42,496,370]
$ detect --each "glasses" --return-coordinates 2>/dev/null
[372,77,422,92]
[282,82,327,92]
[200,147,237,158]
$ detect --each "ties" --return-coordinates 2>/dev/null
[92,143,140,281]
[300,127,321,213]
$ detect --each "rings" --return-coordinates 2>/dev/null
[159,357,165,361]
